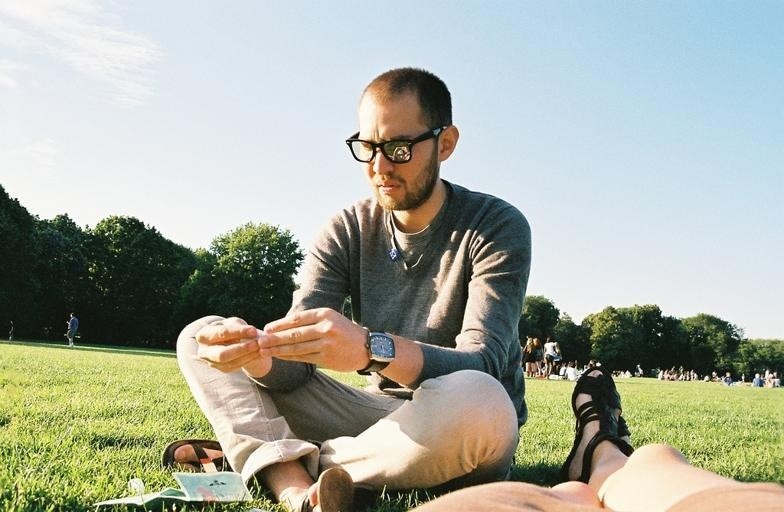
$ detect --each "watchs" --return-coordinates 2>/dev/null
[356,326,396,376]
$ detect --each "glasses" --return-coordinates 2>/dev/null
[346,126,447,163]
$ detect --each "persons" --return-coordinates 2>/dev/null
[520,331,780,388]
[161,65,533,512]
[389,144,410,162]
[408,365,784,512]
[66,313,79,347]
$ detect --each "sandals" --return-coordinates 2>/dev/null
[298,467,355,512]
[161,439,233,472]
[558,366,633,483]
